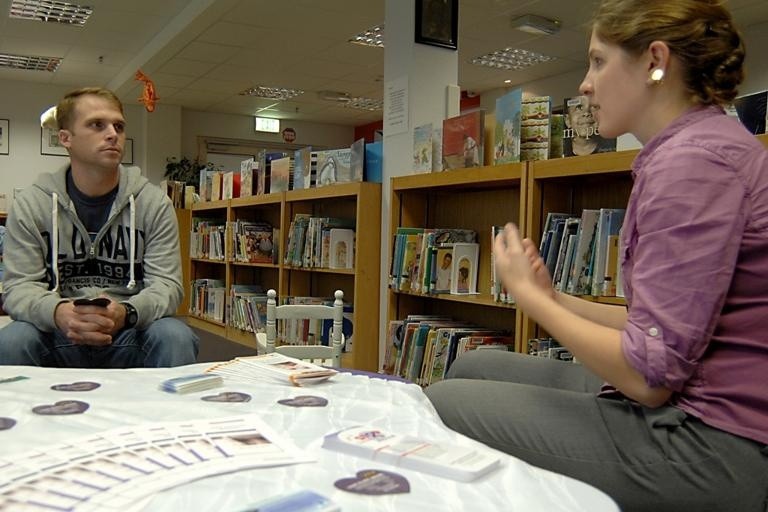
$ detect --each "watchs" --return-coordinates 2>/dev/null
[118,299,139,331]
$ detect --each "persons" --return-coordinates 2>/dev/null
[0,87,200,368]
[422,0,767,512]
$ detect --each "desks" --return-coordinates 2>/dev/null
[0,350,620,512]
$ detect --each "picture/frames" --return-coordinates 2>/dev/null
[121,138,133,165]
[413,0,460,50]
[0,118,9,155]
[40,124,70,158]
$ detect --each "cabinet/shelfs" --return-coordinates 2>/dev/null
[521,133,767,357]
[171,186,383,375]
[382,161,537,393]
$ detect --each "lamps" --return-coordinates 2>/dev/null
[252,116,281,134]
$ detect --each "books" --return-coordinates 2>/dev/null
[539,208,625,298]
[385,315,515,388]
[411,89,643,174]
[387,227,516,304]
[189,212,354,270]
[528,335,582,363]
[160,138,383,209]
[190,279,354,353]
